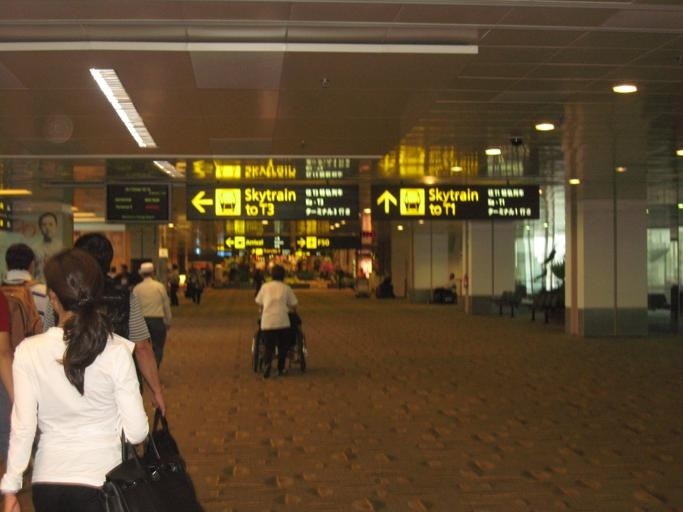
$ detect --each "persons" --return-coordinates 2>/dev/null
[252,264,302,380]
[444,272,456,294]
[356,267,366,288]
[1,231,179,512]
[184,263,213,304]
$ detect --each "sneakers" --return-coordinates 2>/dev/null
[278,368,289,376]
[263,362,271,377]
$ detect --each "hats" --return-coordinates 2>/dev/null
[138,262,154,275]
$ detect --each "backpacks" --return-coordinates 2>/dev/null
[1,278,44,351]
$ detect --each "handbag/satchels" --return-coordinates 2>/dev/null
[97,430,205,512]
